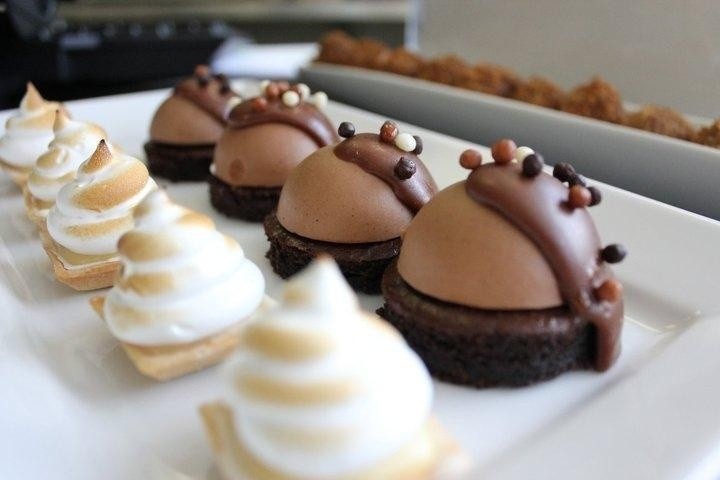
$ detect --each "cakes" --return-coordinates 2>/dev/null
[89,189,271,379]
[0,82,71,189]
[199,257,469,480]
[375,139,627,388]
[262,122,439,296]
[24,109,110,234]
[209,80,340,223]
[144,63,243,180]
[39,140,158,291]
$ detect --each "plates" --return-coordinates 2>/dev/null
[0,58,720,480]
